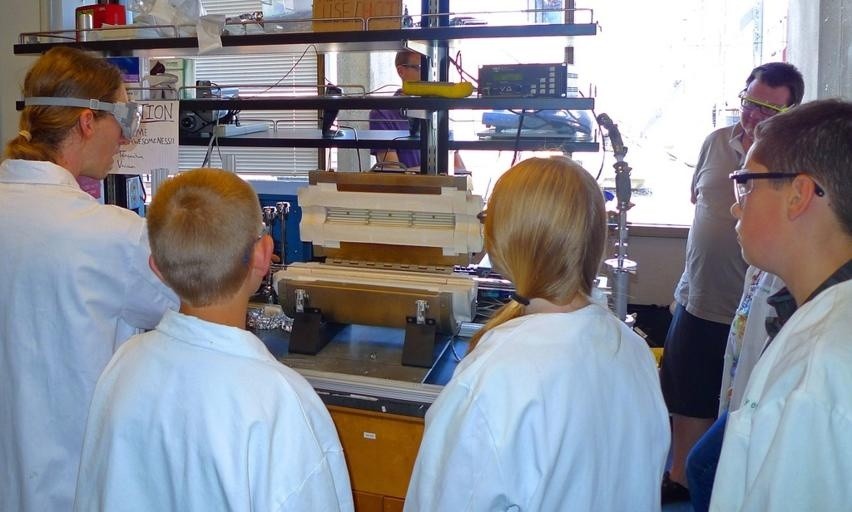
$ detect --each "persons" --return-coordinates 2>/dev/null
[683,266,787,512]
[72,165,357,512]
[657,57,806,503]
[0,43,180,512]
[402,149,673,512]
[366,48,469,175]
[707,96,852,512]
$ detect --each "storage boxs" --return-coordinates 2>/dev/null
[312,0,402,33]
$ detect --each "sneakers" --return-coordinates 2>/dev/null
[662,471,690,502]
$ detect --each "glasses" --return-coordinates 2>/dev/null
[401,63,421,72]
[738,88,795,116]
[477,210,488,224]
[24,97,142,140]
[729,170,824,206]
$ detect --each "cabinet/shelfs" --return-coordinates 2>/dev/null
[326,402,425,512]
[13,20,600,308]
